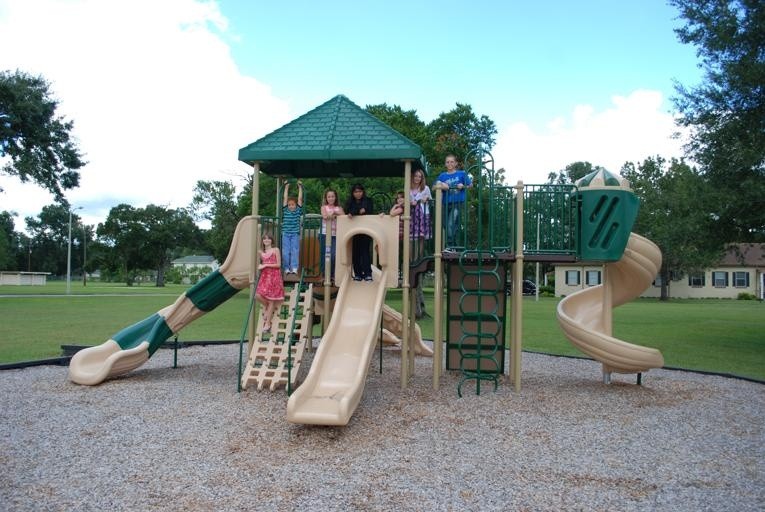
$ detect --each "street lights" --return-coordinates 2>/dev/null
[67,207,84,292]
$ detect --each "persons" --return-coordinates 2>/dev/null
[345,183,373,281]
[318,188,345,280]
[281,180,303,274]
[432,153,473,253]
[379,192,404,275]
[409,168,433,261]
[255,233,285,331]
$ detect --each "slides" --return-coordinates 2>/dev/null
[69,215,261,386]
[285,266,389,426]
[378,303,434,356]
[556,231,665,374]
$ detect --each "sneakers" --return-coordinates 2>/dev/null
[442,248,456,253]
[285,268,297,274]
[354,274,372,281]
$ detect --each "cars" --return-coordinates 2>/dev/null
[506,279,536,296]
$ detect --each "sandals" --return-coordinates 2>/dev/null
[262,312,272,331]
